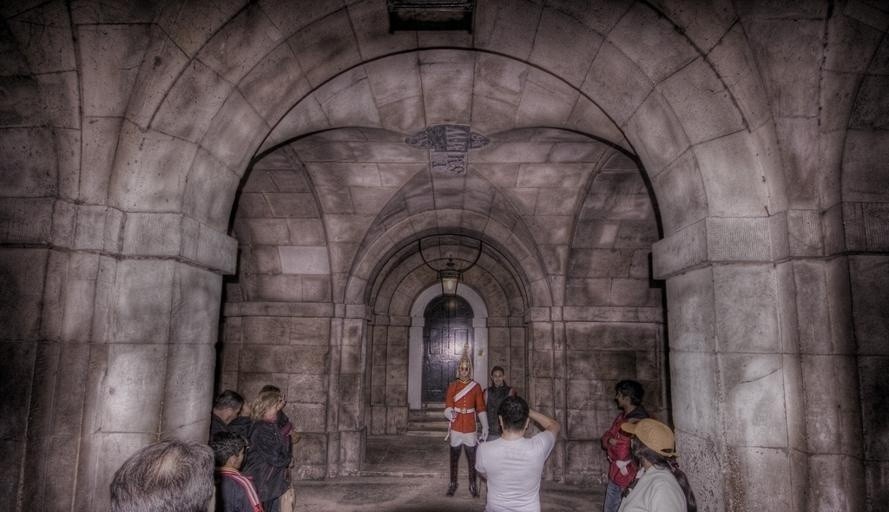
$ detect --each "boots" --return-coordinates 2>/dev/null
[463,444,478,496]
[446,444,462,496]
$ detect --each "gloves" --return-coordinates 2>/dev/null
[443,406,457,423]
[478,411,489,441]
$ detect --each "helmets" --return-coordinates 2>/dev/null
[458,343,471,376]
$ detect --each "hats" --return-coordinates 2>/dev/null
[620,418,680,458]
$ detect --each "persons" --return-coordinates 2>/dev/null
[602,380,656,511]
[442,357,489,498]
[471,394,562,511]
[614,415,696,512]
[476,366,517,443]
[209,385,295,511]
[108,438,216,511]
